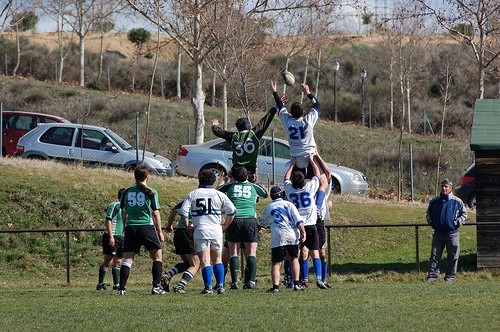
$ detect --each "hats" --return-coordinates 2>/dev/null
[441,179,452,186]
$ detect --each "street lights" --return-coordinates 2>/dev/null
[332,60,340,122]
[360,68,368,126]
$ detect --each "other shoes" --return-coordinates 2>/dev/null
[217,288,224,294]
[445,276,455,283]
[200,288,213,294]
[229,284,239,289]
[294,285,301,290]
[427,276,437,282]
[175,286,186,294]
[249,282,255,289]
[118,287,126,295]
[113,285,119,290]
[301,281,309,290]
[161,274,171,292]
[324,279,333,288]
[267,288,279,293]
[316,280,326,289]
[97,284,106,290]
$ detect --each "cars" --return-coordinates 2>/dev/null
[175,135,368,197]
[453,161,476,210]
[2,110,102,157]
[16,123,175,177]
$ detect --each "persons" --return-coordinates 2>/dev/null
[425,179,467,282]
[259,186,306,293]
[211,94,289,202]
[179,170,237,295]
[117,166,166,294]
[212,166,257,290]
[270,80,322,177]
[283,150,333,290]
[96,187,127,290]
[216,166,268,290]
[161,200,200,294]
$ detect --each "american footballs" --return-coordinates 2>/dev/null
[281,70,295,85]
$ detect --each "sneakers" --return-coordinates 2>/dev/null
[152,286,166,295]
